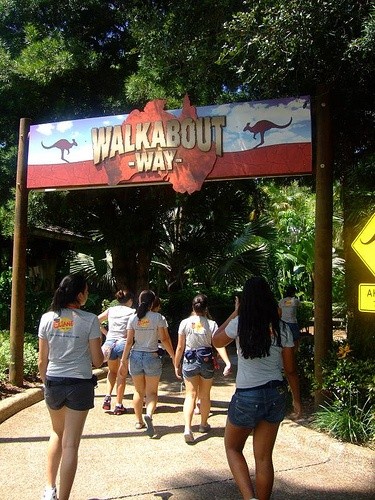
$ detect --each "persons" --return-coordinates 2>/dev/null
[97,290,137,415]
[278,284,300,351]
[121,290,181,437]
[37,275,104,500]
[174,295,230,443]
[213,276,302,500]
[143,297,165,411]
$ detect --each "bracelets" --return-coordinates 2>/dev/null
[170,355,174,358]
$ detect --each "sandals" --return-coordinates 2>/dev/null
[134,421,146,430]
[144,415,155,438]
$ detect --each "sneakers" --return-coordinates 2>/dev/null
[102,396,112,410]
[198,424,210,433]
[113,404,127,415]
[184,430,194,444]
[41,485,58,500]
